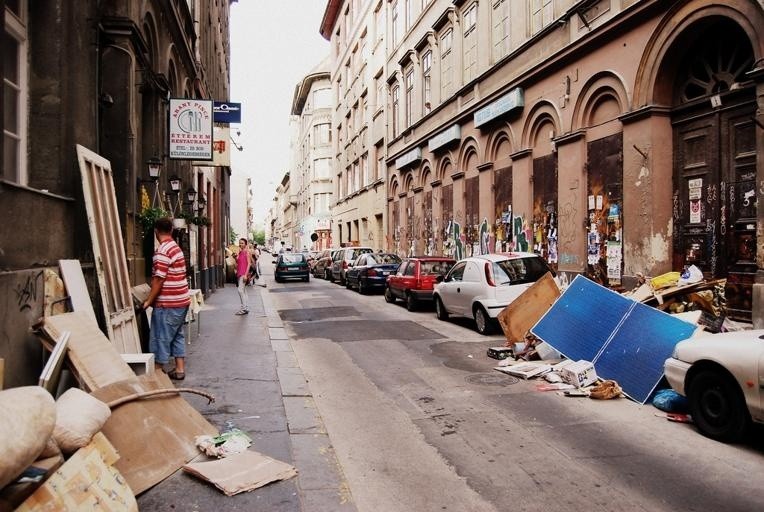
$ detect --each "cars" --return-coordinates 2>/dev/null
[343,251,402,295]
[271,252,311,283]
[302,245,338,281]
[661,325,764,444]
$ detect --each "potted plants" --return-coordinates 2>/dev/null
[172,210,212,231]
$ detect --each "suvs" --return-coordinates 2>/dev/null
[430,250,562,336]
[381,255,459,313]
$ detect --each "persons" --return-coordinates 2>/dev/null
[235,238,252,315]
[253,246,260,275]
[302,245,307,252]
[142,217,191,380]
[255,244,261,275]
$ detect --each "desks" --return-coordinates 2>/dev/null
[181,288,205,345]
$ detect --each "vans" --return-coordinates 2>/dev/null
[329,246,373,287]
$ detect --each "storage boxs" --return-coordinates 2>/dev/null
[563,359,598,389]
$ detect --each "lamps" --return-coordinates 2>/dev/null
[140,156,164,184]
[181,184,197,205]
[164,174,183,195]
[194,194,207,212]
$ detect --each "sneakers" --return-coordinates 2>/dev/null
[168,368,185,380]
[235,309,249,315]
